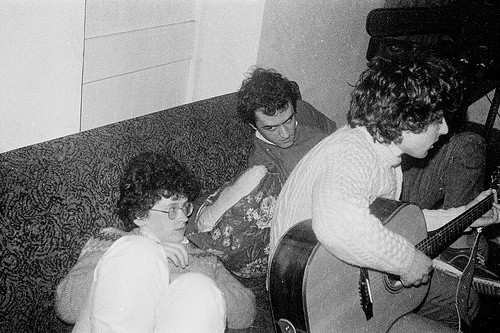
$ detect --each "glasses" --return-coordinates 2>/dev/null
[150,201,196,222]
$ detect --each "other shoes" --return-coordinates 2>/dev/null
[442,247,473,276]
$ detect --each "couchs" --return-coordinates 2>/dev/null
[1,91,274,333]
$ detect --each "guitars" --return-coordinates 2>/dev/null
[267,166,500,333]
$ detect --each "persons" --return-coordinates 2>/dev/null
[53,151,256,332]
[267,41,500,332]
[237,65,338,191]
